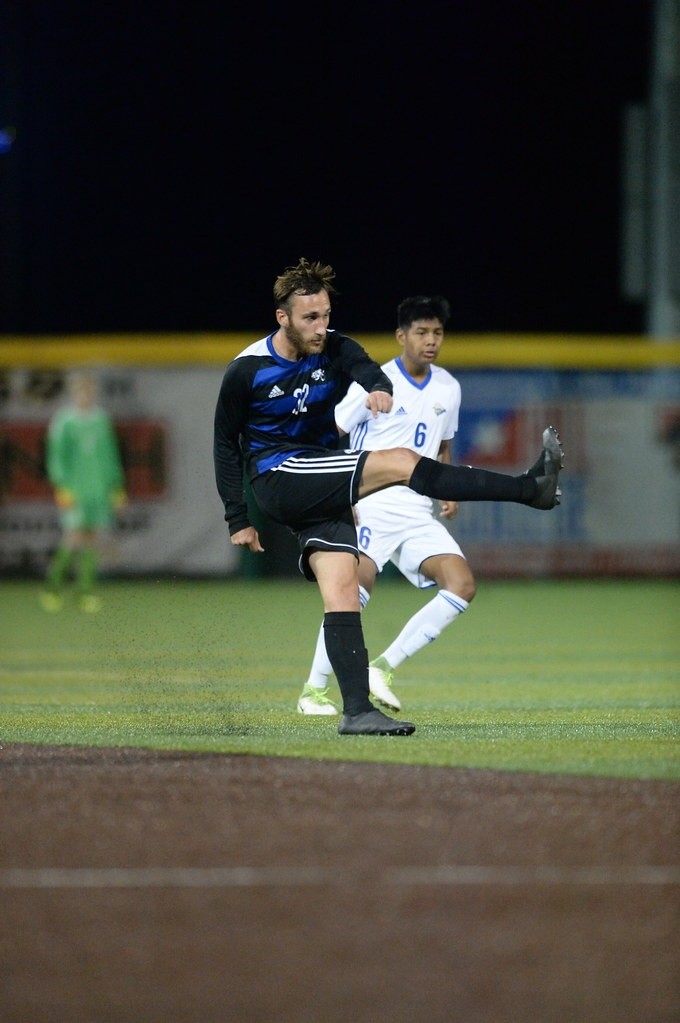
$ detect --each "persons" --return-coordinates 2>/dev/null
[41,376,126,612]
[214,258,565,736]
[298,301,478,714]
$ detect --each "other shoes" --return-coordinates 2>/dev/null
[76,593,99,614]
[40,592,62,612]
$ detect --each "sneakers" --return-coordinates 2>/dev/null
[528,426,565,510]
[296,683,338,715]
[368,668,400,711]
[338,709,415,735]
